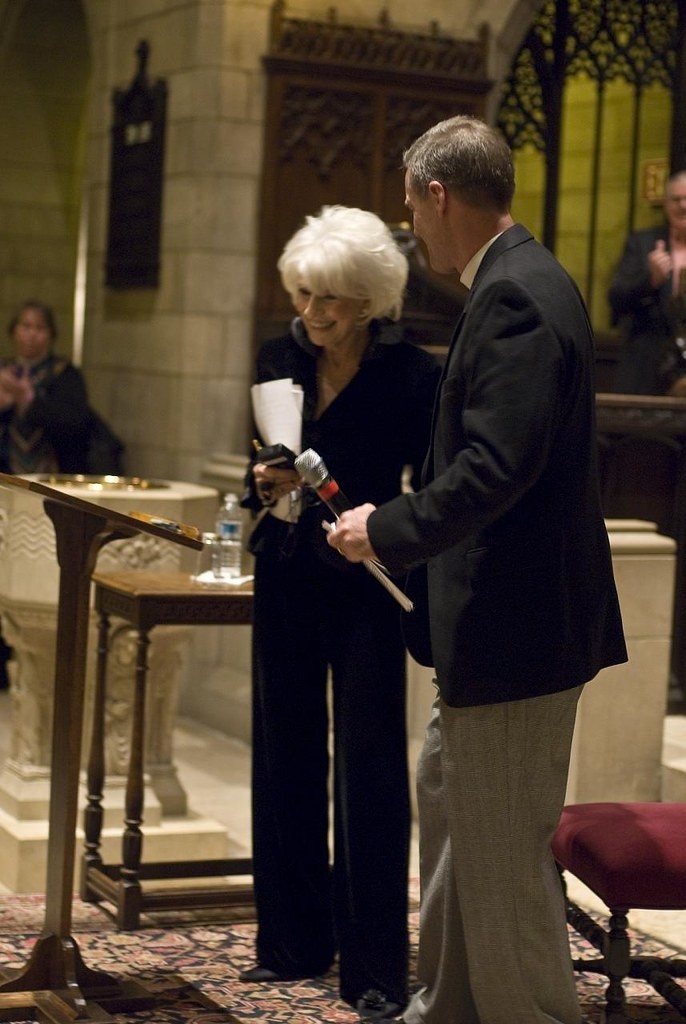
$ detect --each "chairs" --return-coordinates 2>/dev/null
[549,802,686,1024]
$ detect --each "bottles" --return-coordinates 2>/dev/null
[214,493,243,578]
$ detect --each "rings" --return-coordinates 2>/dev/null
[260,482,272,491]
[264,492,272,501]
[338,546,344,555]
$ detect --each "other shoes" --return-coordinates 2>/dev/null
[239,965,318,982]
[358,989,398,1017]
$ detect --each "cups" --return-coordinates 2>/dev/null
[192,534,220,582]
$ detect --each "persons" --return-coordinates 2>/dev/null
[320,117,631,1024]
[605,168,686,399]
[0,302,89,473]
[238,205,442,1009]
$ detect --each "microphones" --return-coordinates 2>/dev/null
[295,448,354,520]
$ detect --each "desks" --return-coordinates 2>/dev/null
[78,569,255,933]
[594,391,686,716]
